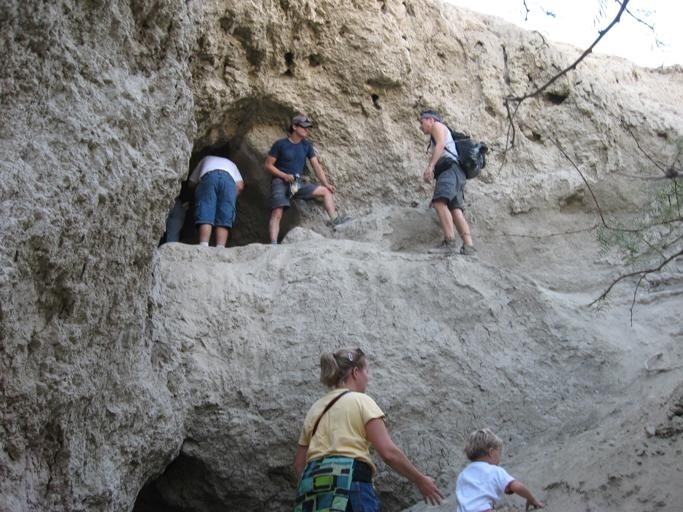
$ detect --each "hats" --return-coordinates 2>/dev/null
[292,116,313,128]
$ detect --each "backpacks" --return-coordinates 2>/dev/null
[431,123,488,178]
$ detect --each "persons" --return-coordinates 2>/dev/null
[419,109,475,255]
[189,147,245,247]
[162,177,190,242]
[265,115,349,244]
[454,428,544,512]
[293,347,445,511]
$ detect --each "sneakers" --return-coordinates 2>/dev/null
[459,245,478,257]
[332,215,354,228]
[427,237,456,253]
